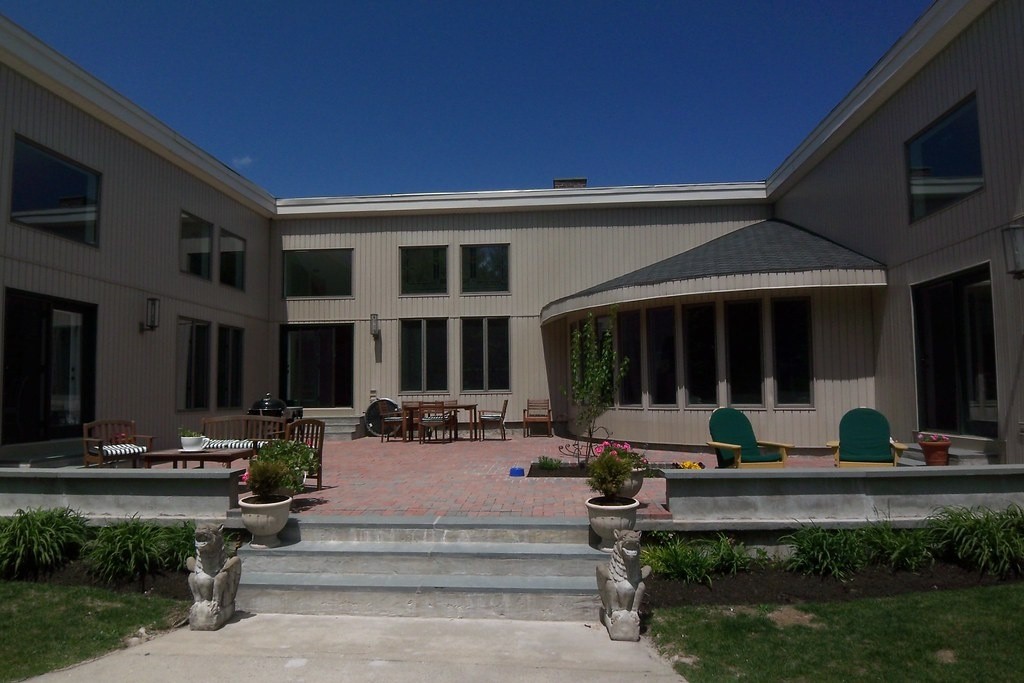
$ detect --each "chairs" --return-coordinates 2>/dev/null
[826,407,908,467]
[478,400,508,441]
[522,398,553,439]
[247,419,325,495]
[706,406,795,469]
[378,399,458,445]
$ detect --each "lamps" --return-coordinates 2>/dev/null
[139,298,160,334]
[369,314,381,340]
[1001,223,1024,274]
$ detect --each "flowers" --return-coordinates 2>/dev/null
[594,441,648,470]
[917,434,950,442]
[241,439,321,493]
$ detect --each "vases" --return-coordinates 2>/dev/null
[271,487,295,498]
[919,442,951,466]
[606,467,647,498]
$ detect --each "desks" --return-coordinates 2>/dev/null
[401,403,478,442]
[140,448,255,469]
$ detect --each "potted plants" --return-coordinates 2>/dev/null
[178,426,211,450]
[237,457,293,549]
[585,459,641,554]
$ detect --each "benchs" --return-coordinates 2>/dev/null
[182,415,287,468]
[83,418,159,468]
